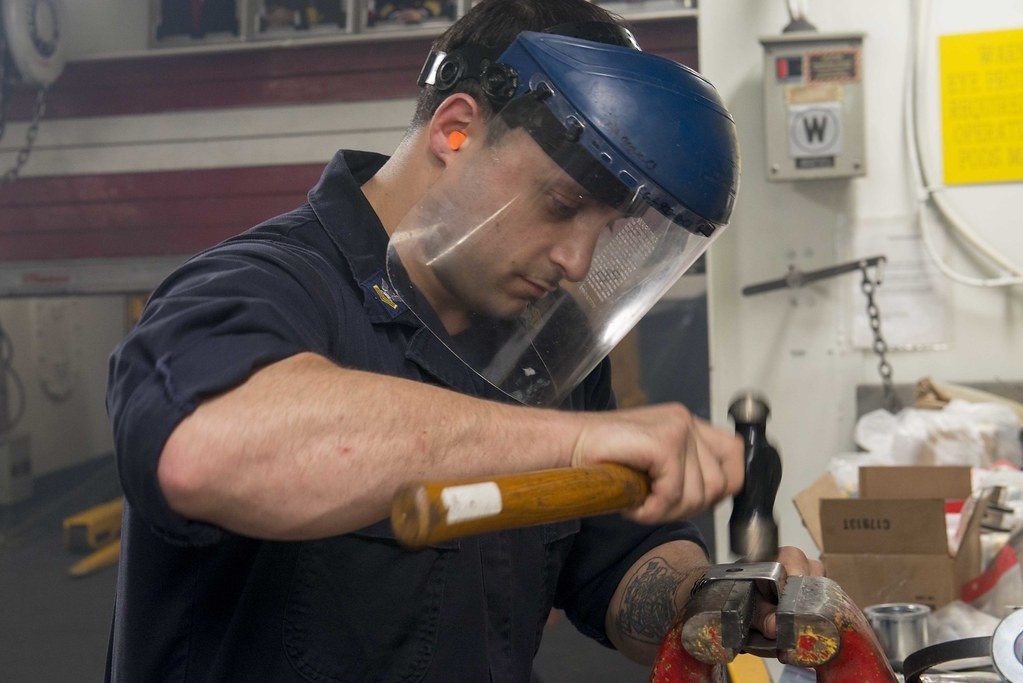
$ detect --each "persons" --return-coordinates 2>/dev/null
[103,0,827,683]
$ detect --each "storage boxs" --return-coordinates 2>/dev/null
[792,465,984,614]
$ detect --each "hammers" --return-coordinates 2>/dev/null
[388,394,786,561]
